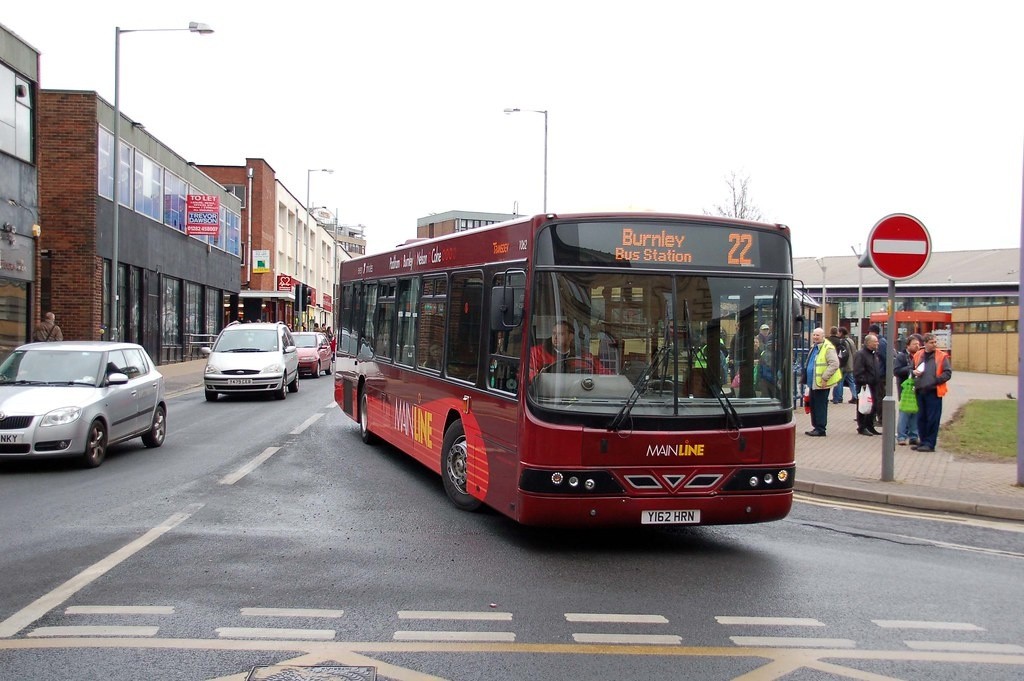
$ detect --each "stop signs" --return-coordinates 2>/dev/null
[866,212,934,281]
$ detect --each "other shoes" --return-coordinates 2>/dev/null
[858,428,873,436]
[871,428,882,435]
[911,444,935,452]
[830,398,843,403]
[909,440,918,445]
[898,439,906,445]
[805,429,826,436]
[848,397,857,404]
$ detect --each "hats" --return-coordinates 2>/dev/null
[868,324,879,334]
[760,324,769,330]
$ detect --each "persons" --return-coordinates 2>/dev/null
[33,312,63,342]
[894,335,920,446]
[302,322,307,331]
[910,333,952,452]
[313,323,333,341]
[529,320,615,385]
[853,335,882,436]
[868,325,887,427]
[694,322,738,398]
[798,328,842,437]
[828,326,856,405]
[423,341,443,370]
[753,323,772,397]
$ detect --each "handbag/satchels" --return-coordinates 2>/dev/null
[899,373,919,414]
[857,384,873,415]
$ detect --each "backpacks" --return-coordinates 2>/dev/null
[836,339,849,367]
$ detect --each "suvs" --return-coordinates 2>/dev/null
[201,319,300,401]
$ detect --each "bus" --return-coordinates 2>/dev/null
[334,211,806,527]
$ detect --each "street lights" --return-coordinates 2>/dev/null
[815,255,831,339]
[502,107,549,214]
[306,168,335,331]
[512,201,520,218]
[850,243,865,350]
[111,22,217,342]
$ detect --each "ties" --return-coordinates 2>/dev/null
[558,352,569,368]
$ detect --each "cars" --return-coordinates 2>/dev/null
[0,340,168,469]
[291,330,333,378]
